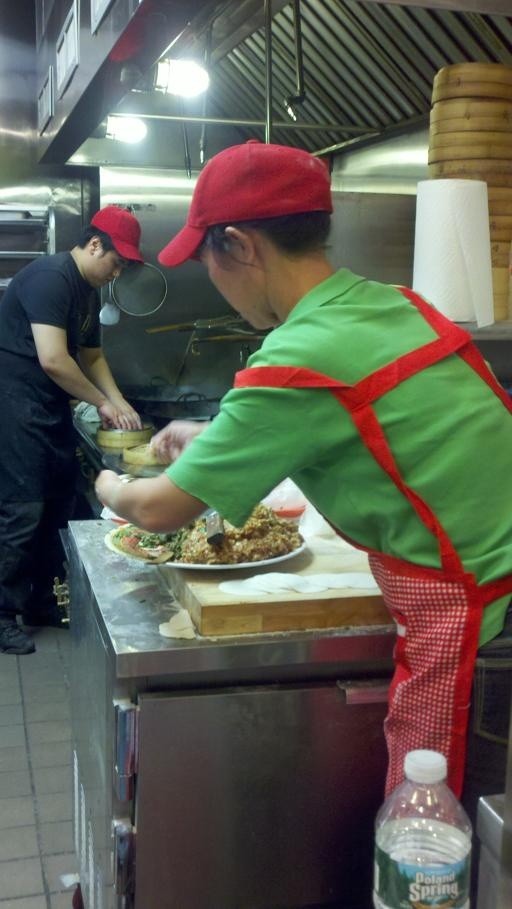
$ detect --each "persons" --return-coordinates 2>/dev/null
[0,206,144,654]
[94,139,512,794]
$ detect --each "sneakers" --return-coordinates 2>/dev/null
[90,206,144,266]
[0,604,68,655]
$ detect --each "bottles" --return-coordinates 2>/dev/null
[369,747,474,909]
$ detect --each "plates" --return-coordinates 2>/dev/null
[103,519,310,572]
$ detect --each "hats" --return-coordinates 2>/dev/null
[156,137,334,267]
